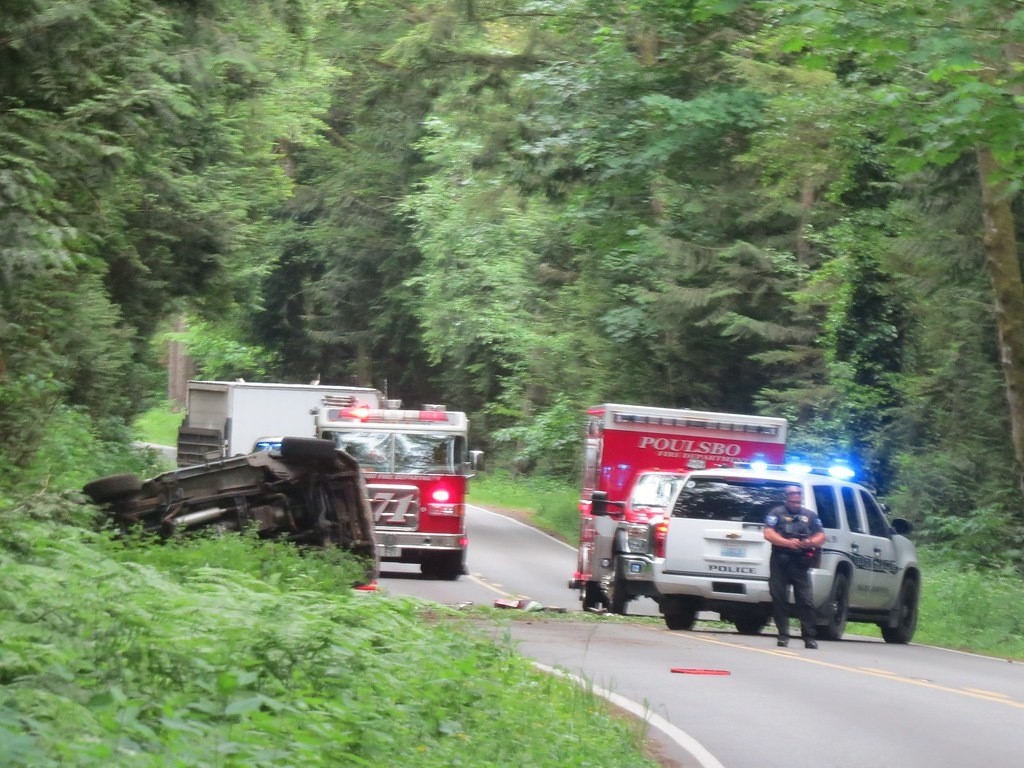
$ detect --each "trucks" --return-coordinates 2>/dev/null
[175,376,405,468]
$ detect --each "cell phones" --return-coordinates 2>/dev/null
[800,534,807,542]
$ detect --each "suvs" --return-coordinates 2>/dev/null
[651,462,922,643]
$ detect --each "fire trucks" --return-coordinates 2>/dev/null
[566,406,789,614]
[310,394,485,581]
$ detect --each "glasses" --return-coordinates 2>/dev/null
[785,491,800,495]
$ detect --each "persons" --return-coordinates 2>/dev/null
[764,486,827,649]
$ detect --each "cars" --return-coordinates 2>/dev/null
[82,436,379,588]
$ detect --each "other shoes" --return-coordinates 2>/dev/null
[777,635,820,650]
